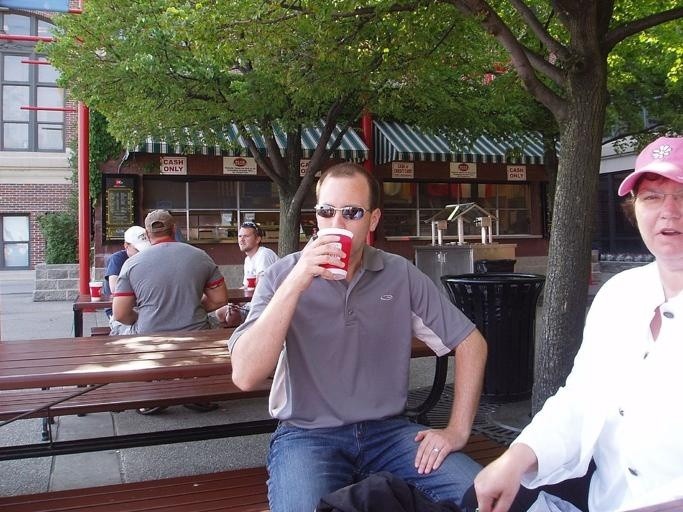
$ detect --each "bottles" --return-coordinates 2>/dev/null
[88,281,104,302]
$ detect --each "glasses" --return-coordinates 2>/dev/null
[637,189,683,210]
[241,222,258,234]
[314,205,371,220]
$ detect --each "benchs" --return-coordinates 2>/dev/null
[0,372,273,421]
[0,434,508,512]
[91,326,111,337]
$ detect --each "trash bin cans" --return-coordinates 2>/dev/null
[441,272,546,404]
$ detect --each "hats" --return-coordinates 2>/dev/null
[124,226,151,252]
[145,210,173,232]
[618,137,683,196]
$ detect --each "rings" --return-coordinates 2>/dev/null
[433,448,441,453]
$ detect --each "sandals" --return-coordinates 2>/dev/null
[184,402,219,411]
[136,407,168,414]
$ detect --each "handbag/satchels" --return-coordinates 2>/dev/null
[313,471,462,511]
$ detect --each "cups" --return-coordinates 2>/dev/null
[244,276,258,291]
[316,227,354,280]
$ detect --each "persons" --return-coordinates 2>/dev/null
[102,226,152,323]
[225,164,489,512]
[214,221,281,327]
[112,209,229,415]
[472,138,683,512]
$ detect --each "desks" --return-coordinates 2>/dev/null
[0,328,236,390]
[73,289,254,338]
